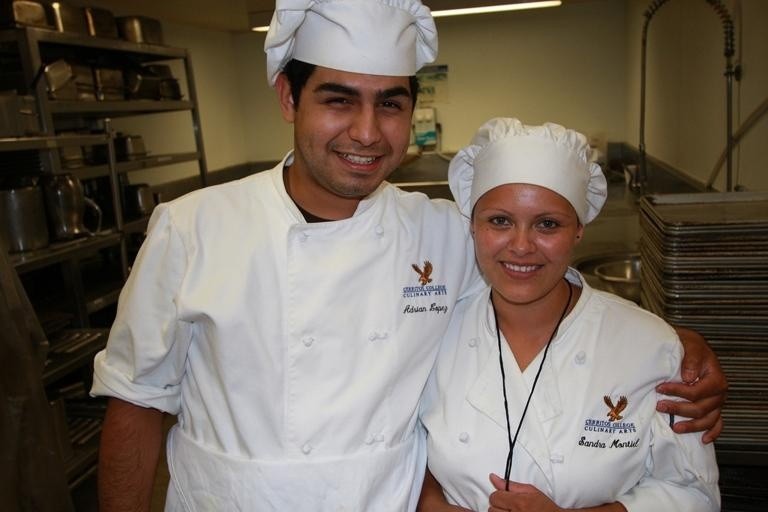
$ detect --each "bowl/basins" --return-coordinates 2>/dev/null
[593,257,641,303]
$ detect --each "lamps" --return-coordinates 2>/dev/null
[249,0,562,32]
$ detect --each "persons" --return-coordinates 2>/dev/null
[85,0,730,512]
[415,113,725,512]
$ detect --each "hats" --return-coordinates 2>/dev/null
[265,0,439,88]
[448,117,607,226]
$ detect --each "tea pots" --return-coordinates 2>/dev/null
[38,169,102,244]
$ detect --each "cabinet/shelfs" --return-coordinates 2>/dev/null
[0,26,211,492]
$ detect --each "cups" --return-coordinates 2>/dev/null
[1,185,49,254]
